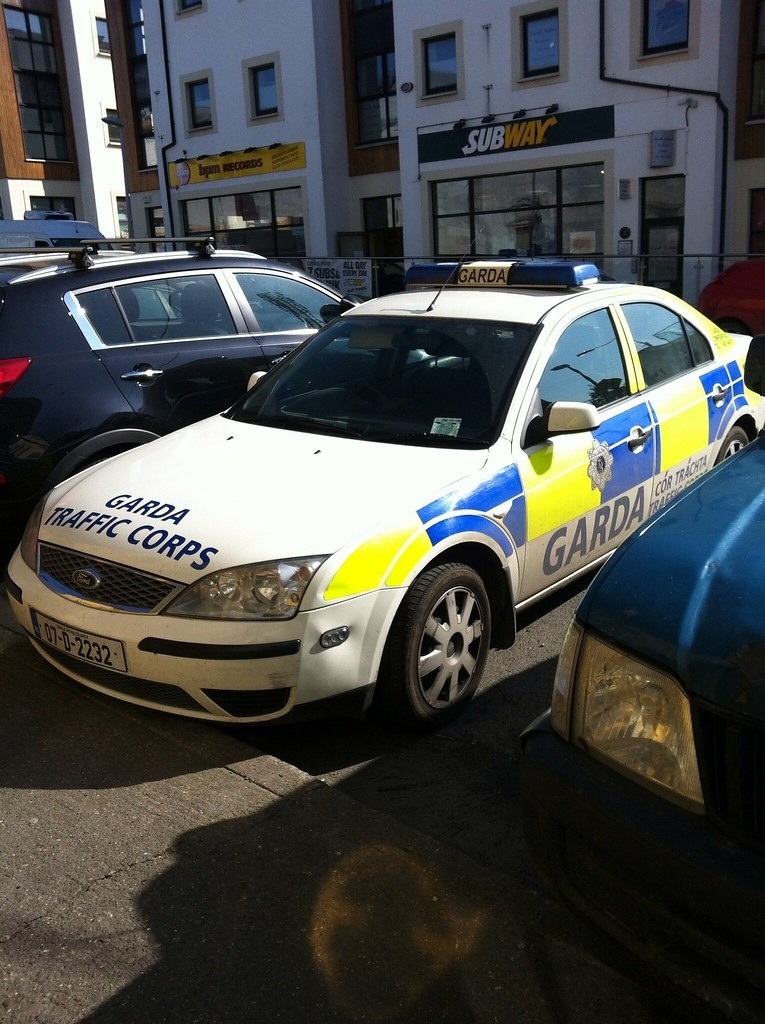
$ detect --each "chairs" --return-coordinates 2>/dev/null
[405,326,484,419]
[92,289,155,344]
[177,283,231,339]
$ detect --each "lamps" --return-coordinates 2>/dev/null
[451,103,560,131]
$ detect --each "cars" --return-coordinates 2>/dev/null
[510,424,764,1024]
[6,257,765,735]
[0,247,195,327]
[696,258,765,337]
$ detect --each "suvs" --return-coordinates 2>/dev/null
[0,234,452,580]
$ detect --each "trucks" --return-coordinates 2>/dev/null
[0,208,114,255]
